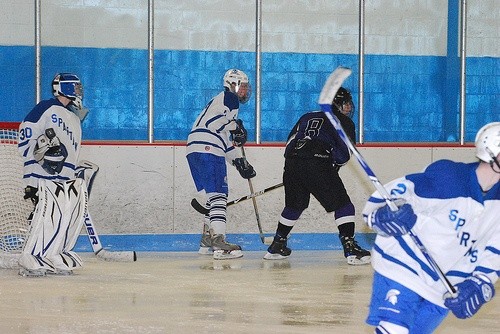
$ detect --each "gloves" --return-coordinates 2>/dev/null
[442,274,495,319]
[333,163,347,171]
[371,198,417,237]
[232,157,256,179]
[229,119,247,147]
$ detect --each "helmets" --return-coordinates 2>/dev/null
[332,87,355,119]
[474,122,500,163]
[52,73,82,110]
[223,69,252,104]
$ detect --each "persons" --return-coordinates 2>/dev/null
[361,121,500,334]
[267,88,370,258]
[185,69,256,253]
[16,72,84,271]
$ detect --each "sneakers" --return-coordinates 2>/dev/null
[263,233,291,259]
[198,224,215,255]
[339,233,372,265]
[209,228,243,260]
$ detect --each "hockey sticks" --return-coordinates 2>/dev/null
[84,209,137,263]
[190,181,286,216]
[240,146,274,245]
[316,67,456,296]
